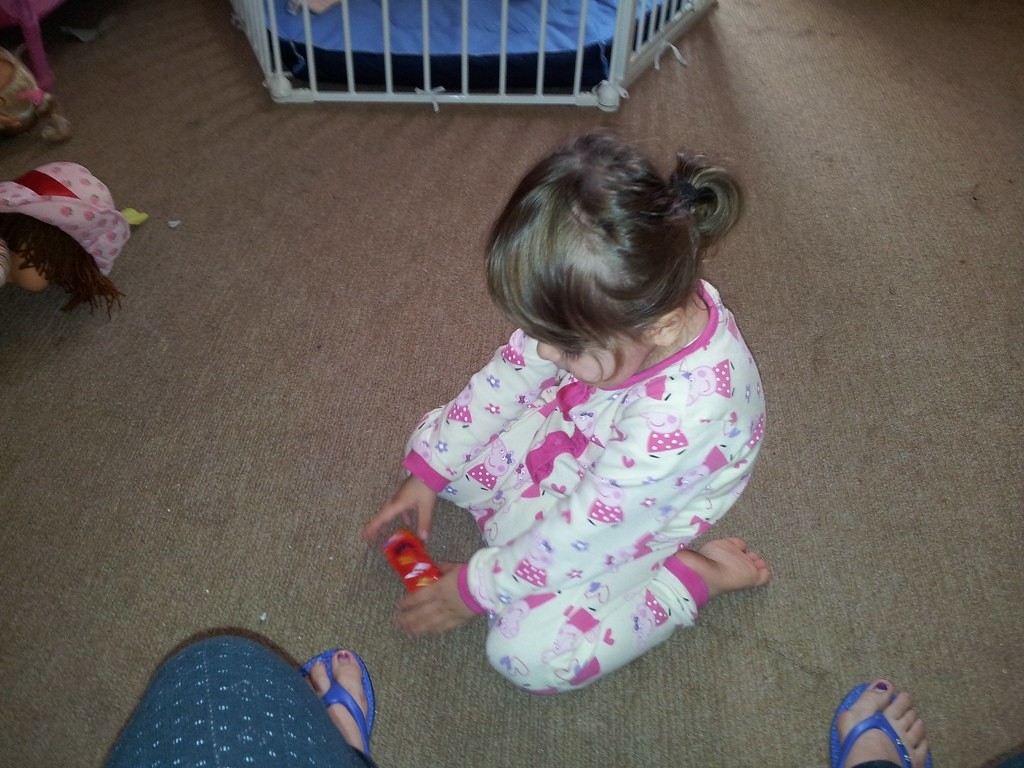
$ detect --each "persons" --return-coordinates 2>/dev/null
[0,45,74,142]
[361,129,771,694]
[0,161,149,320]
[99,634,933,768]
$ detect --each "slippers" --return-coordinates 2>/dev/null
[830,684,933,768]
[300,649,374,759]
